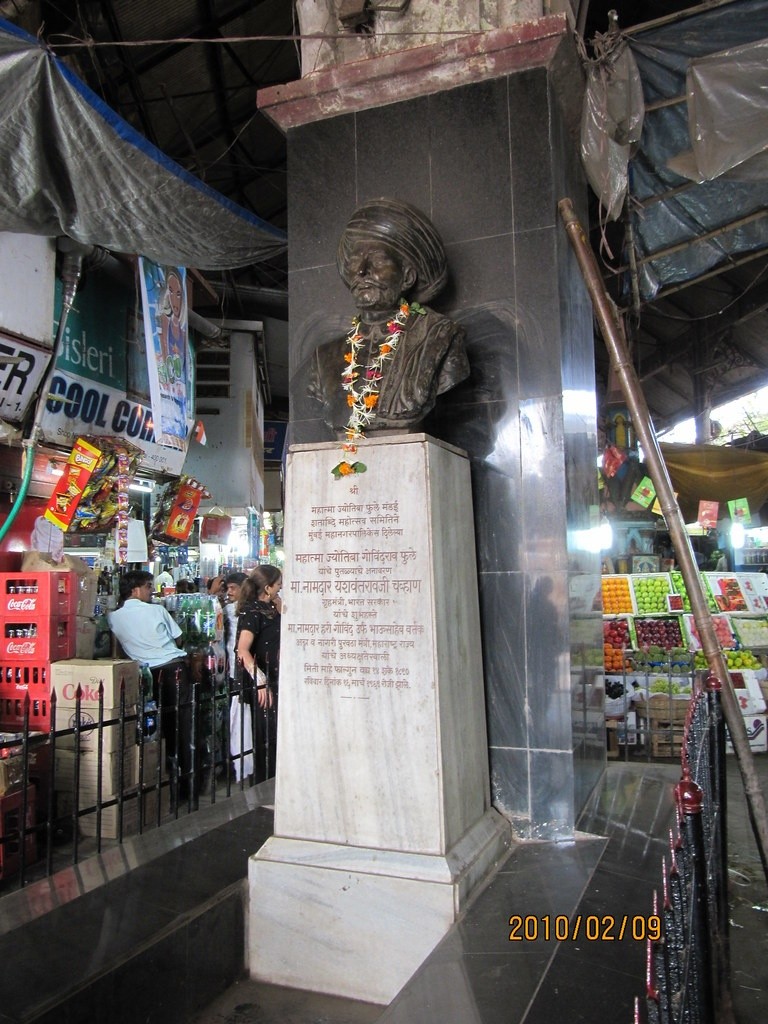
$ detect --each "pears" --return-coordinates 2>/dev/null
[604,643,633,673]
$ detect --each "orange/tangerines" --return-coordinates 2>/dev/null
[694,649,762,670]
[602,578,632,614]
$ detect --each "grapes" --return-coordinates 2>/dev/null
[634,678,691,694]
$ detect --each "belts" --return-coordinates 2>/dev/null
[149,658,184,670]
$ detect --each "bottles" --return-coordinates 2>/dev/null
[140,594,230,737]
[92,563,136,617]
[0,585,65,715]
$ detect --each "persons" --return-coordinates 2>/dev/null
[96,565,280,812]
[308,198,470,440]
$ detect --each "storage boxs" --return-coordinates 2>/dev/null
[142,774,171,825]
[632,554,662,574]
[0,699,55,732]
[0,755,30,795]
[135,738,165,785]
[0,571,78,616]
[0,615,77,661]
[602,572,768,758]
[53,744,139,796]
[0,784,38,880]
[50,658,139,710]
[0,661,52,699]
[76,615,95,659]
[20,550,98,617]
[55,705,137,754]
[26,745,53,778]
[56,783,144,840]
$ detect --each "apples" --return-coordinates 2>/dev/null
[635,620,683,648]
[673,575,715,610]
[603,622,631,649]
[633,577,670,613]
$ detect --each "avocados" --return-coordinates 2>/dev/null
[631,645,690,673]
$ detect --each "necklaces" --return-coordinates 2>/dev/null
[331,299,426,478]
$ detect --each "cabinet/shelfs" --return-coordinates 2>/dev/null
[740,547,768,566]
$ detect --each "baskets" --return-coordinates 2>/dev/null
[633,698,690,718]
